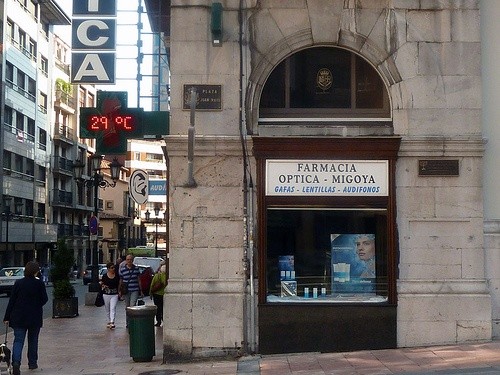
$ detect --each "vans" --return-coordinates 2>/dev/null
[133,257,164,273]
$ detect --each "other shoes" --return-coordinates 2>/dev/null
[13,363,20,375]
[106,323,116,329]
[154,322,161,327]
[29,364,38,369]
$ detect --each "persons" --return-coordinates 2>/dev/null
[102,262,120,328]
[356,234,375,277]
[119,253,144,328]
[43,263,49,285]
[115,258,125,300]
[3,261,48,375]
[289,256,294,271]
[149,265,166,326]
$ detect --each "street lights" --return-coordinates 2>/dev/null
[144,206,166,258]
[72,151,123,293]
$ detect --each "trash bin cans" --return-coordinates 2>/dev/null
[125,306,157,361]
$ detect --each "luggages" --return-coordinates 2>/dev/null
[0,323,12,375]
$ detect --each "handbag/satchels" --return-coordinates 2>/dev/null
[121,283,128,295]
[151,274,164,294]
[95,288,106,307]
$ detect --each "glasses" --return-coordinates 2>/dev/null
[112,266,116,269]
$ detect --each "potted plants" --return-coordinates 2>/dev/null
[48,233,79,317]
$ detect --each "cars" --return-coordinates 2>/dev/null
[0,266,44,295]
[83,263,109,285]
